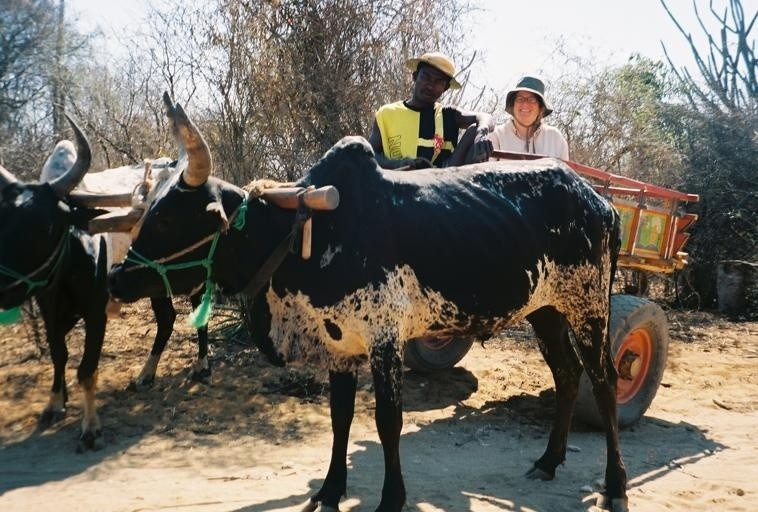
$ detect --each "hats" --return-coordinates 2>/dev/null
[404,52,462,91]
[504,76,553,118]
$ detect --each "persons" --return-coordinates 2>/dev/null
[369,52,495,170]
[479,77,569,164]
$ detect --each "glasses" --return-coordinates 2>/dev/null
[513,96,538,104]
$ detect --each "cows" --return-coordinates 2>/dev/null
[103,88,630,511]
[0,112,213,452]
